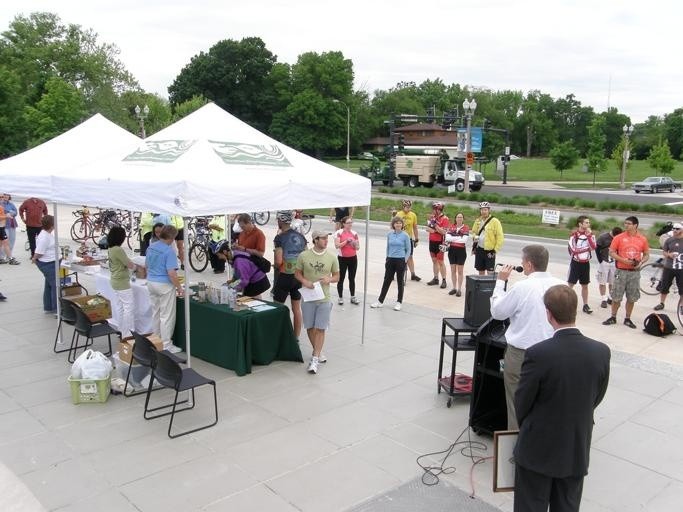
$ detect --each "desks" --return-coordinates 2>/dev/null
[60,252,291,370]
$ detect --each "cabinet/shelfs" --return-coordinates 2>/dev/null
[439,318,478,410]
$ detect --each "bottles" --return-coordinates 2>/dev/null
[223,282,237,309]
[198,282,205,303]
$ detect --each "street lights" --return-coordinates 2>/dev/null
[133,105,148,138]
[620,125,635,188]
[461,98,476,193]
[332,99,350,162]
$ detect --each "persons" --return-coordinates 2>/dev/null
[225,214,267,258]
[594,223,621,308]
[271,213,309,341]
[135,211,227,274]
[0,193,48,265]
[295,230,339,373]
[104,228,138,340]
[371,217,411,312]
[329,207,354,230]
[509,283,611,510]
[490,245,570,435]
[334,219,361,304]
[31,216,63,311]
[602,216,649,330]
[143,226,186,356]
[652,221,682,314]
[423,201,451,288]
[445,213,469,298]
[390,198,420,281]
[566,216,597,317]
[209,240,271,298]
[473,201,504,273]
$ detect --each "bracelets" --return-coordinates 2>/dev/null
[176,285,181,289]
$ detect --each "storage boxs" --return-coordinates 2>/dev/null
[66,332,162,405]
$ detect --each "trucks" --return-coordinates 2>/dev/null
[395,154,485,191]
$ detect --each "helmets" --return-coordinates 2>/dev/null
[438,244,450,251]
[401,199,412,207]
[276,210,293,224]
[210,239,230,255]
[433,202,443,210]
[479,201,489,209]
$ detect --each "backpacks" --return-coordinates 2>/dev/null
[656,222,672,236]
[232,252,271,274]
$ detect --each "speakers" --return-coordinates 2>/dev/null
[463,274,507,327]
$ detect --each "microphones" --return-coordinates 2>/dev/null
[497,263,523,273]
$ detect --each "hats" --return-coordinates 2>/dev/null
[312,229,332,239]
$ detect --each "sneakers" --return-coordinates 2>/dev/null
[306,356,319,374]
[337,273,462,311]
[583,297,637,329]
[9,257,20,265]
[163,342,183,354]
[654,303,664,310]
[319,354,327,363]
[0,258,7,264]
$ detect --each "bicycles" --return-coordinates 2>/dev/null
[638,262,682,327]
[70,208,313,271]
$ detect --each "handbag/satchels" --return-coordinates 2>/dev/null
[644,313,677,337]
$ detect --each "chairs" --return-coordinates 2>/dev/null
[53,285,118,362]
[123,328,218,439]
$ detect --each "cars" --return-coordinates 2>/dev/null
[633,177,679,193]
